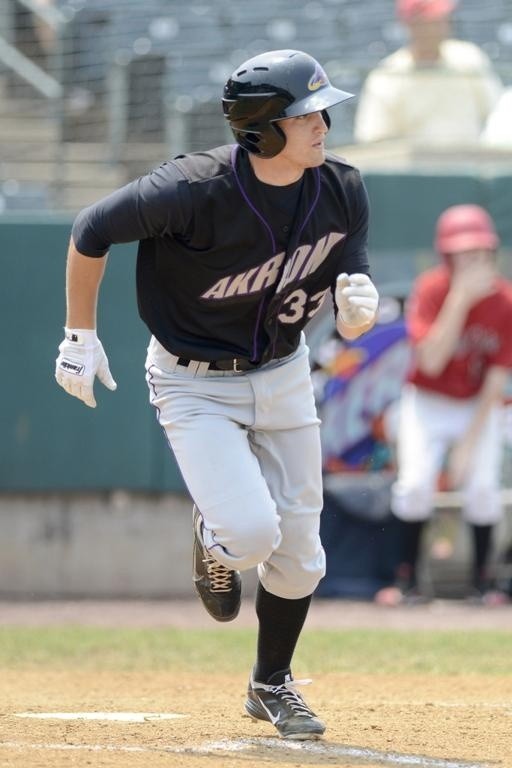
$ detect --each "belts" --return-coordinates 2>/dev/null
[177,356,259,373]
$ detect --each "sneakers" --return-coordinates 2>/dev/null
[244,667,326,740]
[192,505,242,622]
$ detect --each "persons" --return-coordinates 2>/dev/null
[355,0,512,180]
[373,202,512,609]
[53,50,381,737]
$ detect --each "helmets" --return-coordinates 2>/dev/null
[222,49,356,158]
[435,203,499,253]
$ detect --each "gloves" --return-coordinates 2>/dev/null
[55,327,117,408]
[335,272,379,329]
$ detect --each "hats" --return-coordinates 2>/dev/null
[396,1,455,22]
